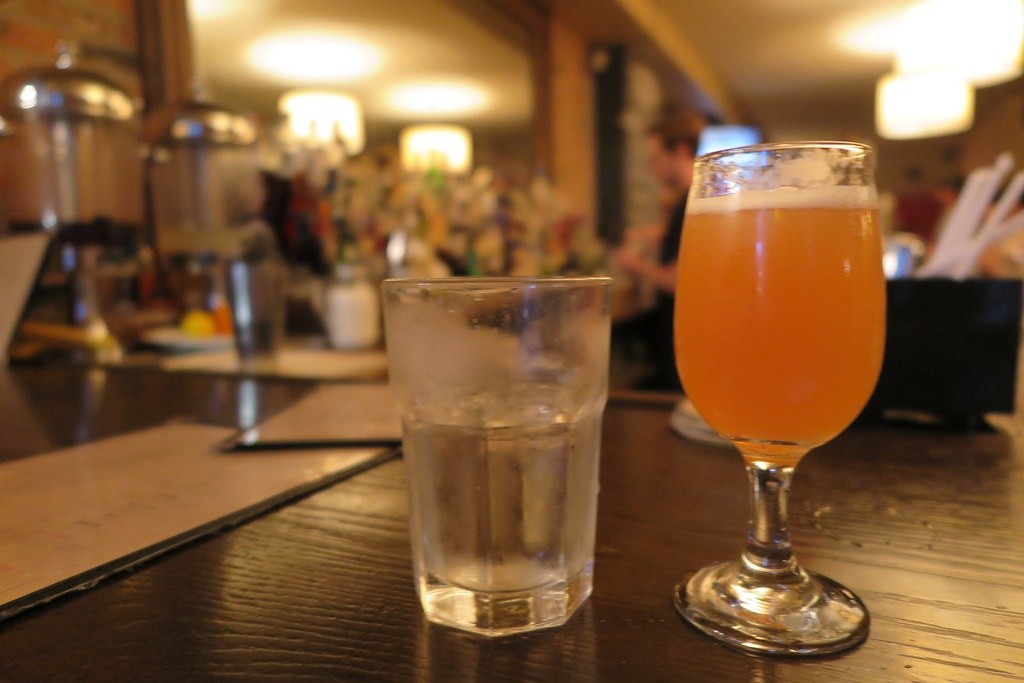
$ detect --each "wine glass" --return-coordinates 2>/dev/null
[672,139,885,658]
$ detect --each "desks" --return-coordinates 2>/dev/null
[0,324,1024,683]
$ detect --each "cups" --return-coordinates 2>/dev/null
[379,273,612,639]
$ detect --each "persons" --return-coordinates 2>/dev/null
[609,104,710,392]
